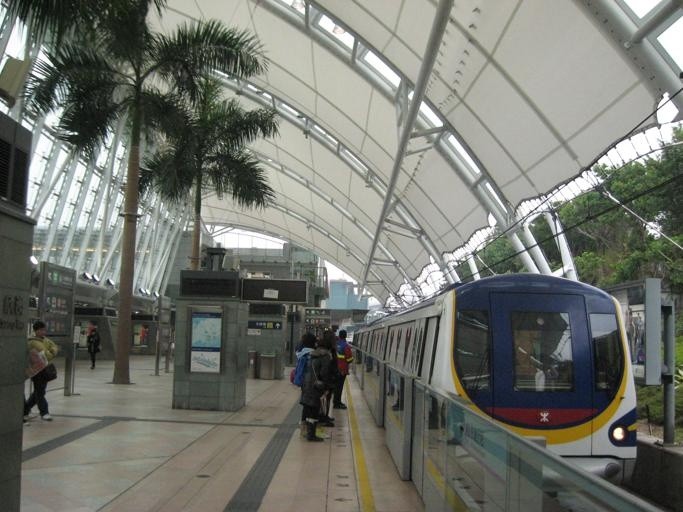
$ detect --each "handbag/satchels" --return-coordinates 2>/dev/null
[315,380,326,389]
[290,368,296,384]
[46,363,57,382]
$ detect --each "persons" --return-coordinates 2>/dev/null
[87,329,100,369]
[628,311,645,365]
[23,321,59,423]
[292,328,354,443]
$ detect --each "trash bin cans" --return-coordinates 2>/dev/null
[248,350,281,380]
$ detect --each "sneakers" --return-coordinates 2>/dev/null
[301,417,335,443]
[334,403,347,409]
[42,413,53,420]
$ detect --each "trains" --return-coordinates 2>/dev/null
[353,272,637,497]
